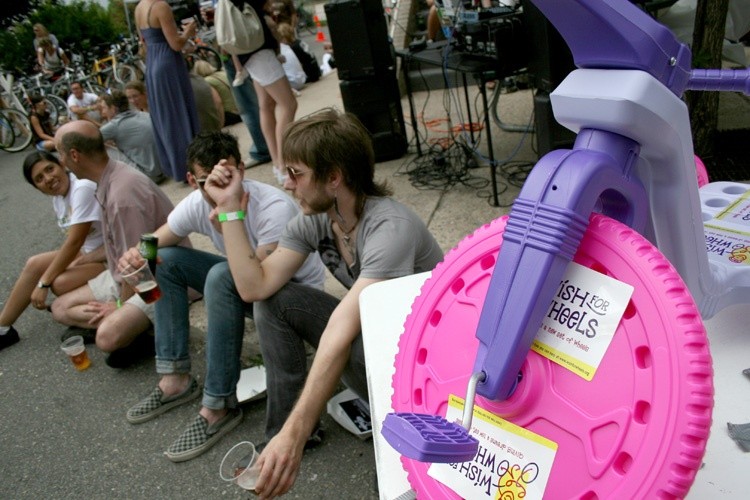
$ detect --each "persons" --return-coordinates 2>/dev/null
[116,130,325,462]
[27,0,321,185]
[426,0,445,43]
[0,151,108,348]
[51,120,200,370]
[204,107,444,500]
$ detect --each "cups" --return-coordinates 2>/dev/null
[120,258,162,305]
[220,441,280,500]
[60,335,91,370]
[181,18,198,36]
[199,1,215,24]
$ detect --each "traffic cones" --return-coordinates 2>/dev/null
[314,16,325,41]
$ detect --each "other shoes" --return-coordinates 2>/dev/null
[273,167,288,185]
[61,327,95,344]
[0,326,19,350]
[167,404,243,461]
[106,335,155,369]
[126,375,201,423]
[244,157,260,168]
[238,424,326,468]
[233,68,248,87]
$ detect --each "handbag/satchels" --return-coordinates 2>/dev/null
[214,1,264,55]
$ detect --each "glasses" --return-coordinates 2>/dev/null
[192,175,206,191]
[287,167,313,184]
[107,88,113,99]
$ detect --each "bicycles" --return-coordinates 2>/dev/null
[0,31,222,153]
[295,2,316,38]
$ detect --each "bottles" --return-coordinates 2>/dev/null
[138,234,159,279]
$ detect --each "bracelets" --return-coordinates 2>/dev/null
[219,211,244,222]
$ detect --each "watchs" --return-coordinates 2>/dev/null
[38,281,52,288]
